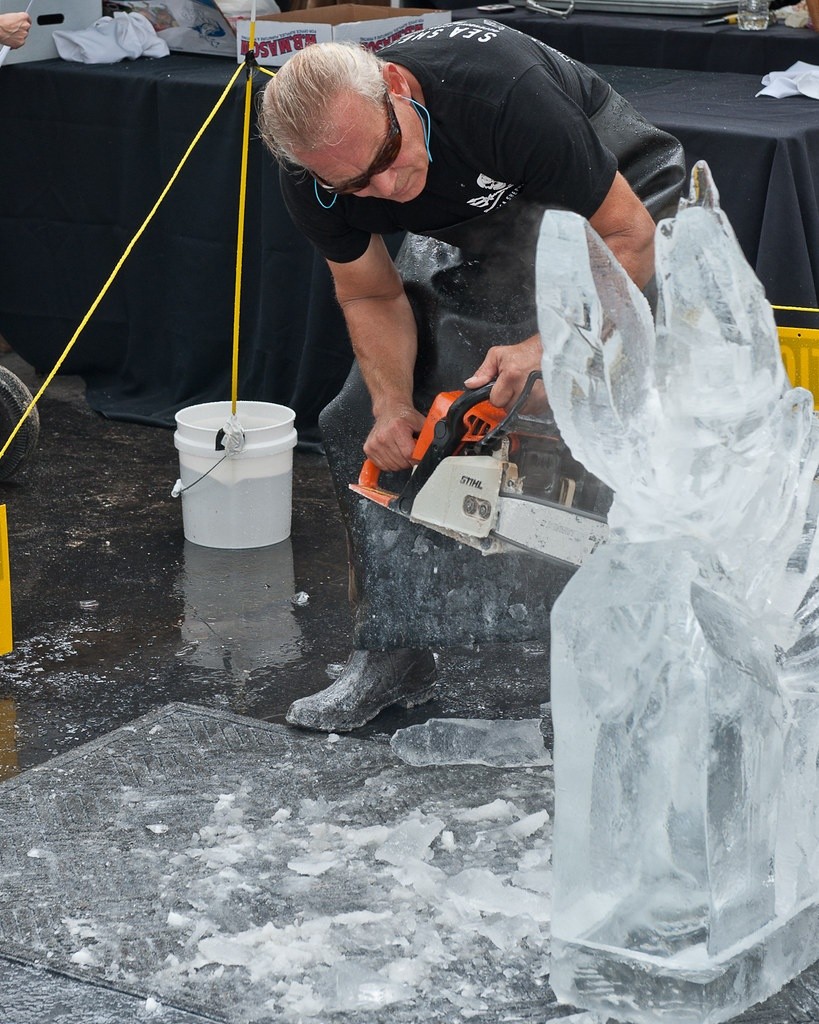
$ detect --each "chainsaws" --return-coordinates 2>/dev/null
[348,380,607,571]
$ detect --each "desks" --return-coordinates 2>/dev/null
[0,0,819,456]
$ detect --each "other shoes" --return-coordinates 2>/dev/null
[287,643,437,732]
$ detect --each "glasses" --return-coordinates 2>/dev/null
[309,87,403,197]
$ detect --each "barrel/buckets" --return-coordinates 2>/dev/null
[172,401,298,549]
[180,540,303,671]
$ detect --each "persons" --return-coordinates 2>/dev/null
[0,12,32,49]
[257,18,687,740]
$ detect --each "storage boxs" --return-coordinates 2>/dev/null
[236,3,452,68]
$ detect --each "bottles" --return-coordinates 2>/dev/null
[737,0,769,30]
